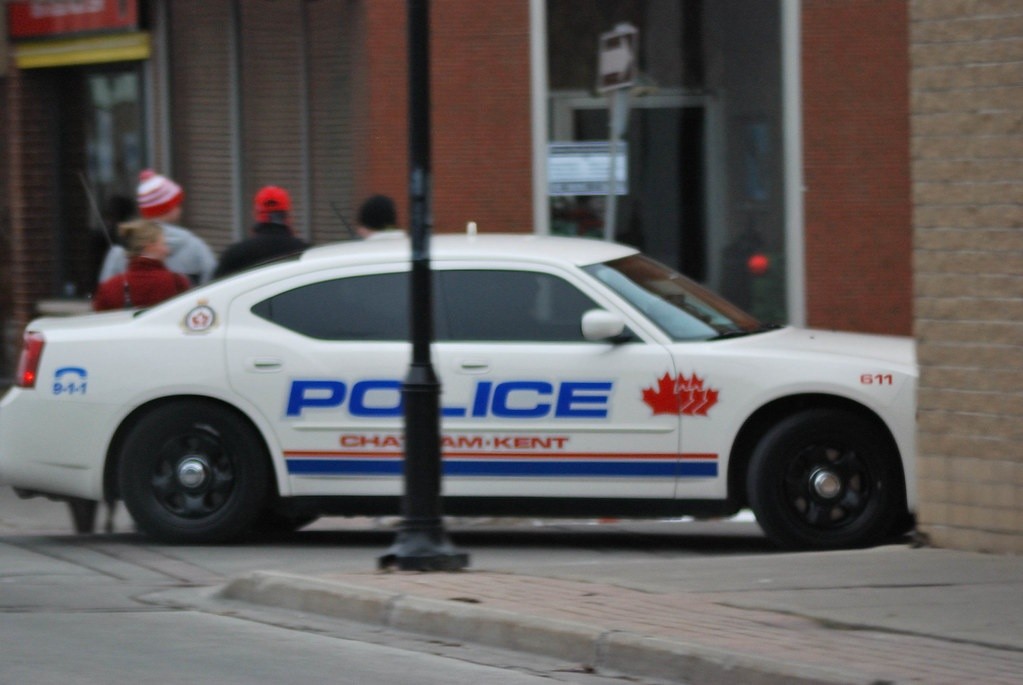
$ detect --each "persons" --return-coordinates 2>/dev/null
[99,172,214,281]
[94,220,189,311]
[353,195,398,239]
[213,186,314,280]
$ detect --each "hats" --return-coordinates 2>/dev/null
[136,170,185,219]
[255,186,291,227]
[118,219,164,256]
[358,196,395,231]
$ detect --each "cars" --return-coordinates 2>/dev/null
[0,223,923,556]
